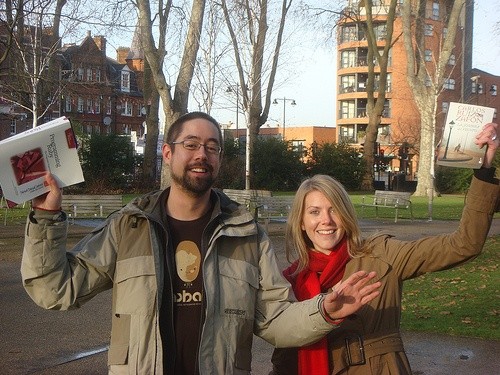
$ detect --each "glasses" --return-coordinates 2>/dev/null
[173,140,220,154]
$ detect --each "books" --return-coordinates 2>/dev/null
[0,116,85,209]
[435,102,494,169]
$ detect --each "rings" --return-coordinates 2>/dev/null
[491,134,497,140]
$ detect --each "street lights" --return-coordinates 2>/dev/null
[273,97,296,141]
[443,120,455,159]
[227,82,249,138]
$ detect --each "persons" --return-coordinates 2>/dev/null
[267,123,500,375]
[20,112,383,375]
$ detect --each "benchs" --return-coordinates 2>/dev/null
[221,189,294,237]
[61,194,123,236]
[362,190,413,223]
